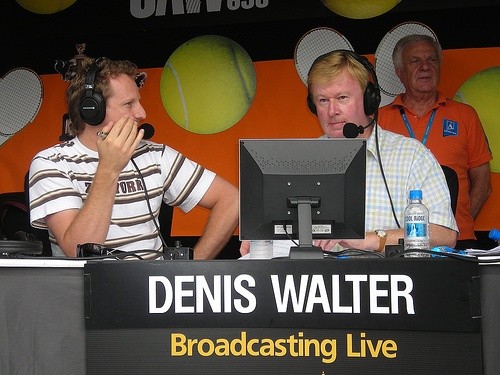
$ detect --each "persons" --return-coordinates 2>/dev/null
[373,34,492,249]
[29,57,239,260]
[239,50,460,257]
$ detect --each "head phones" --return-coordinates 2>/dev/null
[306,49,381,116]
[80,57,106,126]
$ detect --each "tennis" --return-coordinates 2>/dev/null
[16,0,79,14]
[159,34,258,135]
[453,65,500,174]
[321,0,401,20]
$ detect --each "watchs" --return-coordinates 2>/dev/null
[374,230,387,251]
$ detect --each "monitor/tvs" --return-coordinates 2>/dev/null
[238,137,367,261]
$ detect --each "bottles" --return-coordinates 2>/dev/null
[403,190,431,258]
[250,241,273,260]
[489,229,500,246]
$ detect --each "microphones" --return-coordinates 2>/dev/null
[343,109,378,138]
[136,123,155,139]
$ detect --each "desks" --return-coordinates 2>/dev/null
[0,254,500,375]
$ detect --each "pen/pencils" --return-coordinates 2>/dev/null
[96,131,108,138]
